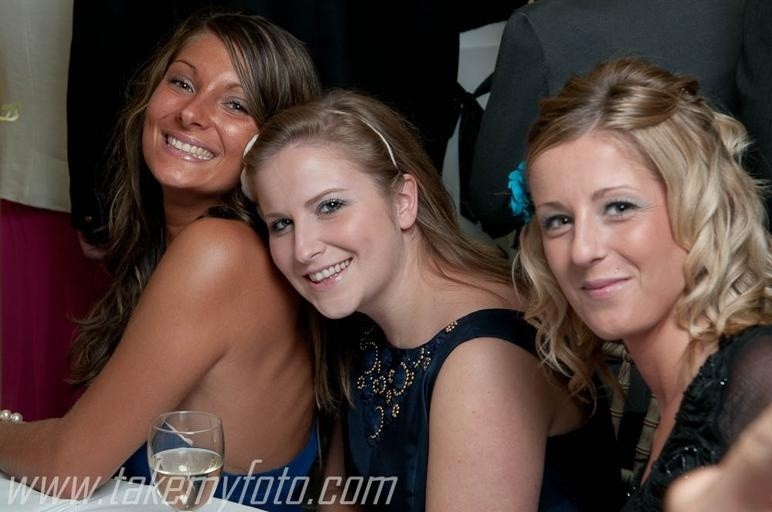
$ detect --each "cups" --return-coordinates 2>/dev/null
[147,411,225,511]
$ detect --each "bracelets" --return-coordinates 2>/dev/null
[1,409,24,424]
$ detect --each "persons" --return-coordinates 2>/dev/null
[505,57,772,512]
[231,91,628,511]
[1,8,335,511]
